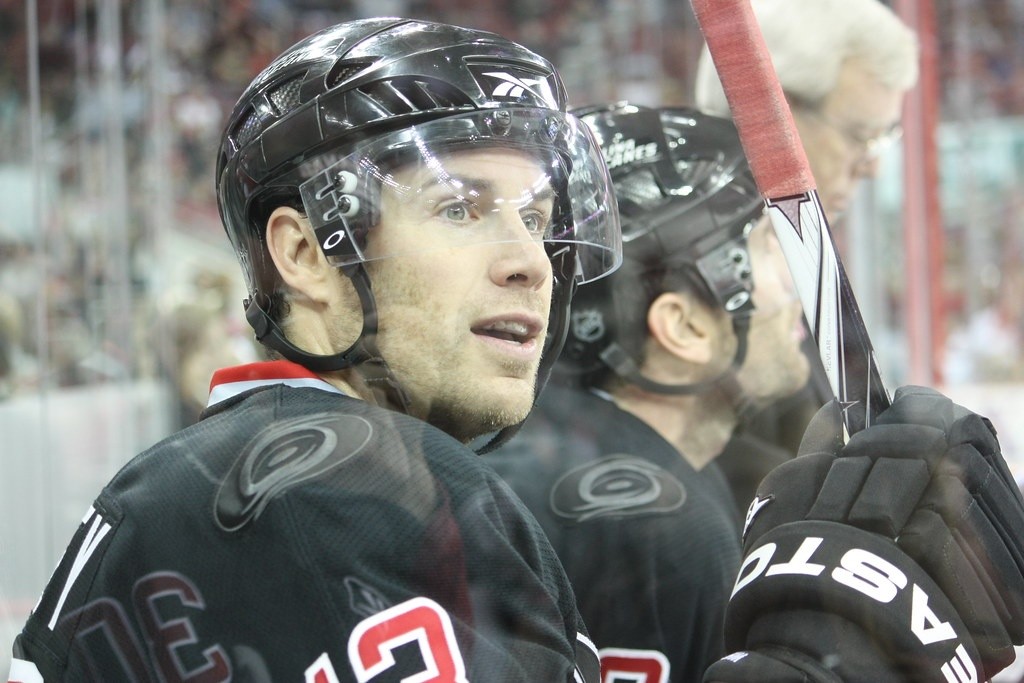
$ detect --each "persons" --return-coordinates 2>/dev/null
[8,15,1024,683]
[455,92,812,682]
[694,0,921,518]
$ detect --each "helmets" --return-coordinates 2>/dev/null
[555,99,770,373]
[216,16,622,328]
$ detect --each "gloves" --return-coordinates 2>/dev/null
[701,384,1023,682]
[711,379,825,522]
